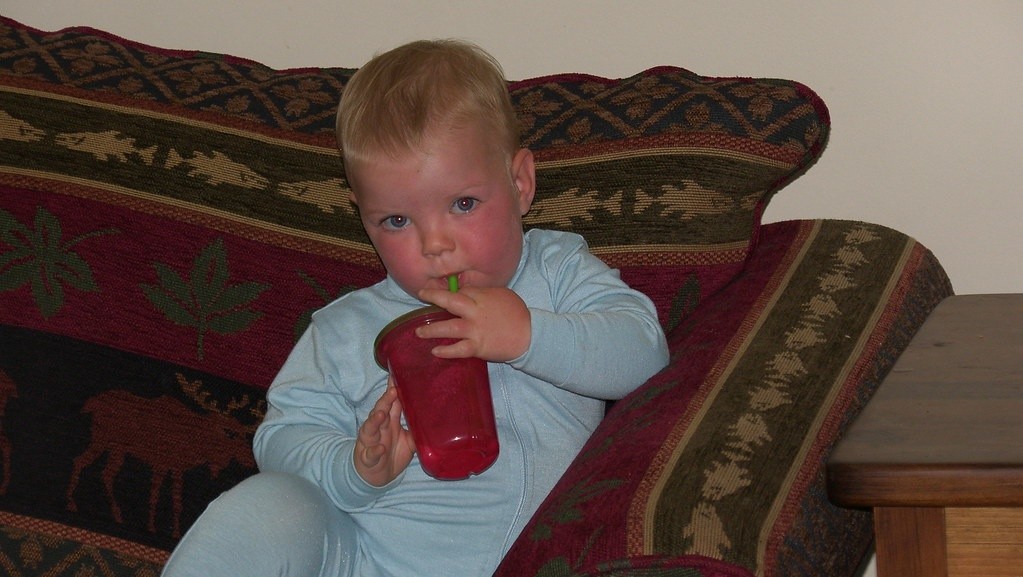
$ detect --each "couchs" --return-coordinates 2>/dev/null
[0,14,953,577]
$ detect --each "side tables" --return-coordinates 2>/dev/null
[829,293,1022,577]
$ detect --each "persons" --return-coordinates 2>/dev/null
[160,37,672,577]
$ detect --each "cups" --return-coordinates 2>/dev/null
[372,303,501,482]
